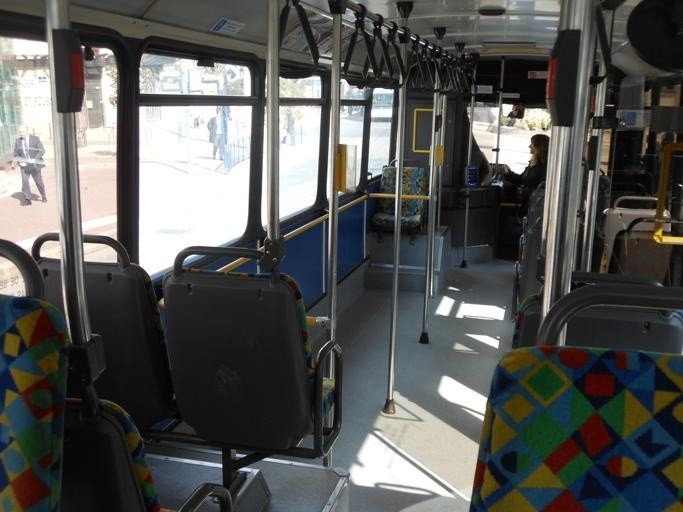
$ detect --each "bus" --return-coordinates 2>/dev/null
[347,86,395,119]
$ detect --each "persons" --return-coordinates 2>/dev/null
[13,124,49,207]
[222,106,233,146]
[208,105,225,163]
[655,131,675,154]
[280,107,296,145]
[494,132,549,262]
[506,105,523,120]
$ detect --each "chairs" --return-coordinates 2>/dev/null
[1,233,343,511]
[468,179,681,511]
[373,159,426,241]
